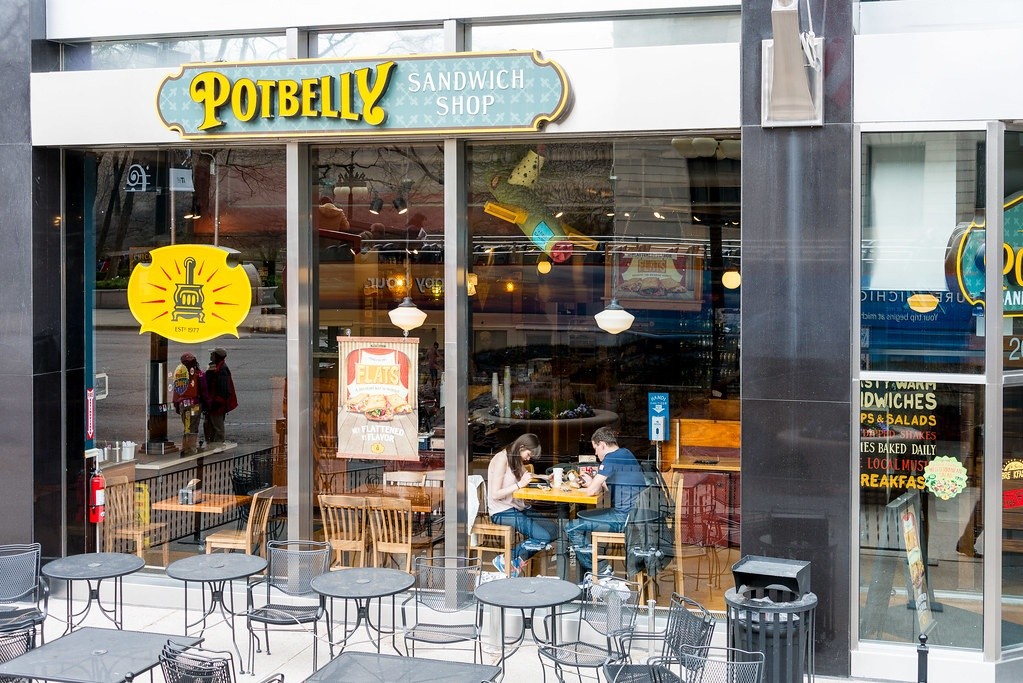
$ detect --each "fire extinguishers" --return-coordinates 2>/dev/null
[89,467,106,523]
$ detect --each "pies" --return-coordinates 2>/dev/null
[621,277,688,295]
[345,393,413,420]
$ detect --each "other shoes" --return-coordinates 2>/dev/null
[957,540,982,559]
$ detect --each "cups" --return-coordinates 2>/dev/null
[113,449,122,463]
[553,468,563,488]
[103,447,111,461]
[122,444,135,460]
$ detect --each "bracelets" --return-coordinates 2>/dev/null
[517,483,521,489]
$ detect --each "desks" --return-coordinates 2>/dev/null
[311,567,417,658]
[0,627,205,683]
[301,649,502,682]
[153,492,253,559]
[43,553,147,632]
[347,482,444,555]
[515,476,605,573]
[476,576,582,682]
[167,553,268,675]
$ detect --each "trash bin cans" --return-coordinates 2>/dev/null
[724,554,819,682]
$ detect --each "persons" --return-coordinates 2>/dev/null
[488,433,557,578]
[565,426,647,588]
[171,347,238,458]
[955,491,983,559]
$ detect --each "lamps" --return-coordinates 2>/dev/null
[393,197,407,216]
[537,250,552,274]
[722,261,740,290]
[908,289,939,314]
[596,248,634,335]
[387,243,428,337]
[368,196,383,214]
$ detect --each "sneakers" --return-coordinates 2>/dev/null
[511,556,528,573]
[607,565,615,575]
[577,569,612,589]
[492,554,517,578]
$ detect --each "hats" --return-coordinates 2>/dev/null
[208,346,227,357]
[181,353,195,363]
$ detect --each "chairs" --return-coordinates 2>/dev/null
[103,476,170,567]
[403,559,483,663]
[250,540,333,671]
[539,474,766,683]
[382,471,427,530]
[365,497,437,587]
[468,474,516,579]
[159,642,285,683]
[319,495,364,568]
[206,485,278,577]
[0,542,52,664]
